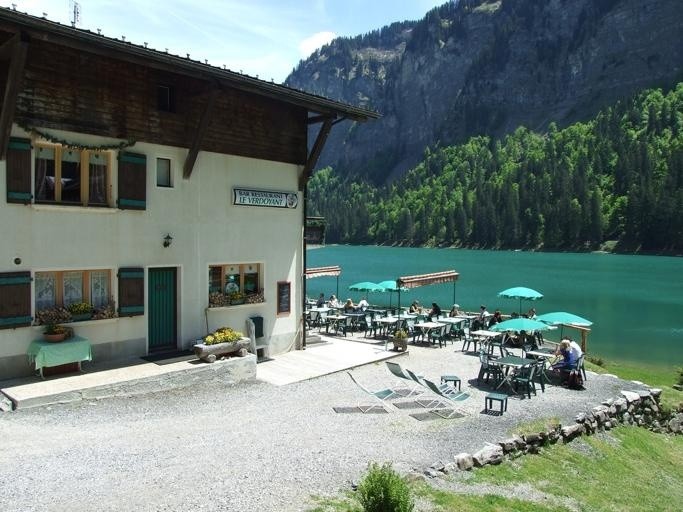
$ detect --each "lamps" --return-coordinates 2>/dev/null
[161,232,174,250]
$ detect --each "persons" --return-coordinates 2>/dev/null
[224,280,239,294]
[448,304,461,317]
[341,299,355,312]
[554,340,575,388]
[561,335,582,361]
[426,301,441,321]
[470,304,537,332]
[409,300,422,313]
[503,330,537,350]
[355,294,369,312]
[327,294,338,308]
[315,291,325,307]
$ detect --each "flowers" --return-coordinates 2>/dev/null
[34,296,115,344]
[200,324,241,346]
[208,286,263,309]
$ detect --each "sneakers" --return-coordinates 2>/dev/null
[556,382,569,388]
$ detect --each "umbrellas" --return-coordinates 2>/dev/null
[487,316,550,378]
[534,311,594,343]
[496,285,544,317]
[346,280,385,304]
[376,279,409,308]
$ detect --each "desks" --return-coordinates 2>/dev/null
[24,334,94,380]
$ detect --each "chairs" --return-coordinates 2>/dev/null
[307,287,598,416]
